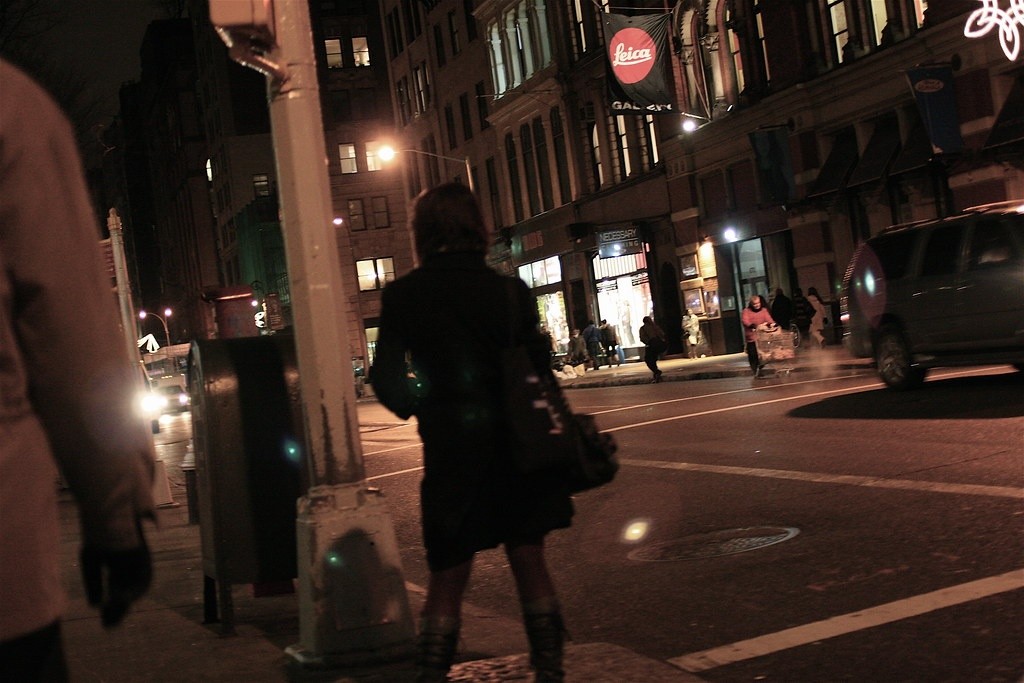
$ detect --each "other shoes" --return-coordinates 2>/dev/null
[595,365,601,369]
[608,364,612,368]
[652,371,662,383]
[617,363,621,366]
[819,336,828,349]
[688,352,699,360]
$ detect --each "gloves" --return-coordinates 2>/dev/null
[823,317,827,324]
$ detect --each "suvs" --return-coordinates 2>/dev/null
[840,200,1024,387]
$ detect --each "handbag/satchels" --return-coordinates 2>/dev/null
[536,364,620,499]
[649,335,668,354]
[681,329,689,337]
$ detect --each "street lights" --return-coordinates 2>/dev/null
[139,309,172,346]
[376,146,474,191]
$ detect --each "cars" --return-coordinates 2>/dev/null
[156,384,188,414]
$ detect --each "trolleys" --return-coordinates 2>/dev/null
[751,328,794,379]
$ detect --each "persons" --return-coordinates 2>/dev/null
[542,326,563,372]
[371,183,564,683]
[566,319,620,372]
[681,308,699,360]
[640,317,663,383]
[741,287,829,377]
[0,56,156,683]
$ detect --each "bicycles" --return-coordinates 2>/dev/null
[791,321,800,351]
